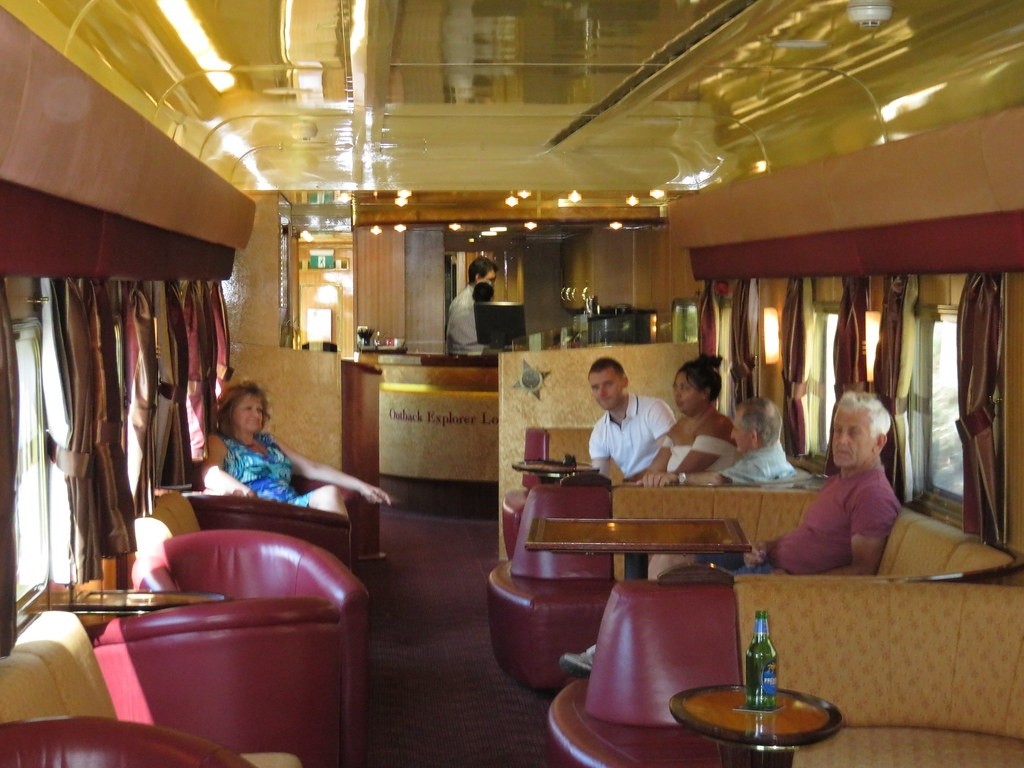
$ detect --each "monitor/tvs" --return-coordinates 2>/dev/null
[473,300,527,351]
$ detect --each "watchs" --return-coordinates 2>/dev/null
[678,471,687,484]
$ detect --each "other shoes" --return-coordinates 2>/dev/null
[560,652,591,679]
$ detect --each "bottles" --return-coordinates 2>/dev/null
[746,611,777,711]
[373,330,381,346]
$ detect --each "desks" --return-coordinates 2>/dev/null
[525,518,756,584]
[81,591,227,642]
[673,684,843,768]
[512,459,602,485]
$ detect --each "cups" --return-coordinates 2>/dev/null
[362,338,370,345]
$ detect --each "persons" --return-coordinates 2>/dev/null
[198,379,391,562]
[446,255,504,356]
[625,353,739,487]
[560,390,903,679]
[587,356,677,487]
[634,397,797,487]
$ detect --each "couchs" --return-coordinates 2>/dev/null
[503,426,826,559]
[546,581,1024,768]
[487,487,1024,699]
[0,467,370,768]
